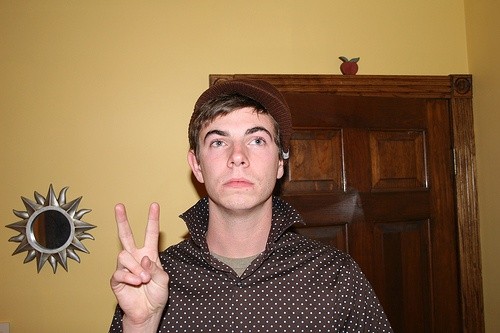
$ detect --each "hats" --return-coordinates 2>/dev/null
[193,80,291,166]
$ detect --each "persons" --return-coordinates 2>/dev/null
[107,78,394,333]
